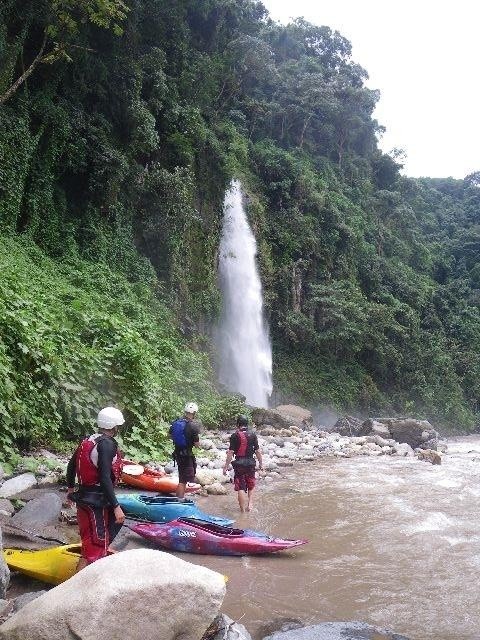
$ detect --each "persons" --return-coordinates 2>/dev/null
[63,406,126,575]
[168,401,203,502]
[223,413,265,518]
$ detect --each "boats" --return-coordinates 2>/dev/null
[124,516,311,557]
[7,543,122,584]
[115,494,237,529]
[119,458,200,493]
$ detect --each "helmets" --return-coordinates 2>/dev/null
[237,414,247,423]
[185,402,199,414]
[97,407,125,430]
